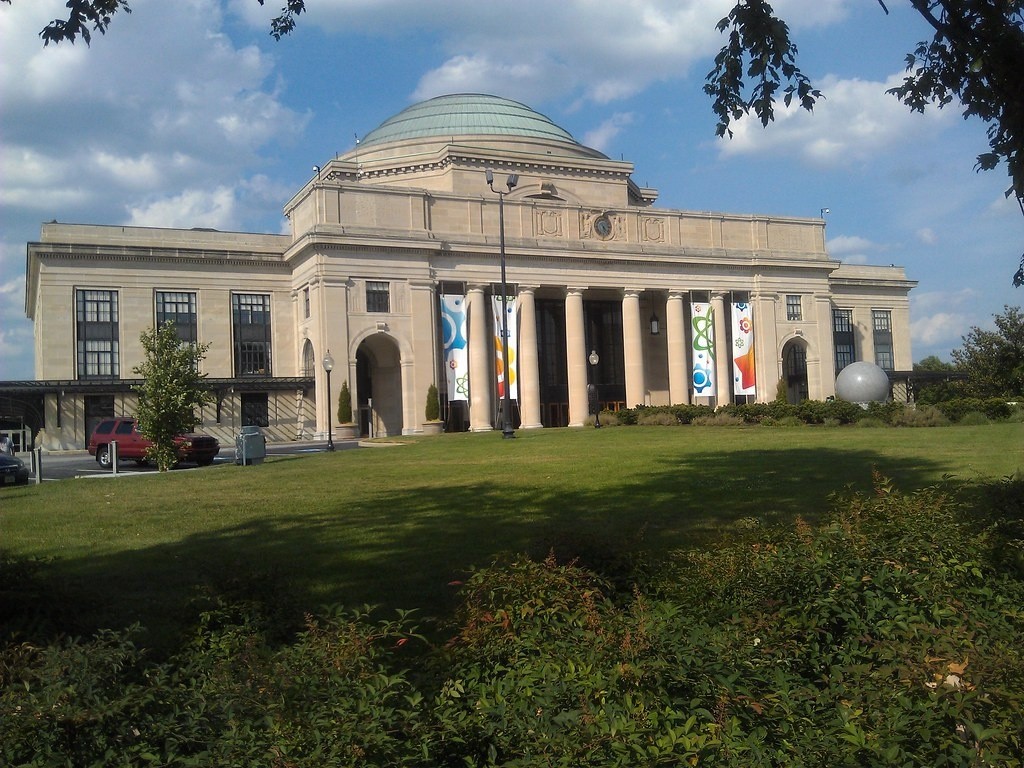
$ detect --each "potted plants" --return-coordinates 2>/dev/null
[422,384,445,437]
[335,381,359,438]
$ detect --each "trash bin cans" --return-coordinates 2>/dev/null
[235,426,266,466]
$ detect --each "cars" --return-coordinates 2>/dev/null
[0,449,29,488]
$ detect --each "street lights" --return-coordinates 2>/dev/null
[589,350,602,428]
[323,348,336,451]
[485,169,519,438]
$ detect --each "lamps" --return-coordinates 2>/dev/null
[649,313,659,335]
[589,350,600,365]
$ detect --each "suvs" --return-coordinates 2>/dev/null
[89,416,221,471]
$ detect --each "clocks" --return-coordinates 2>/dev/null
[595,216,612,237]
